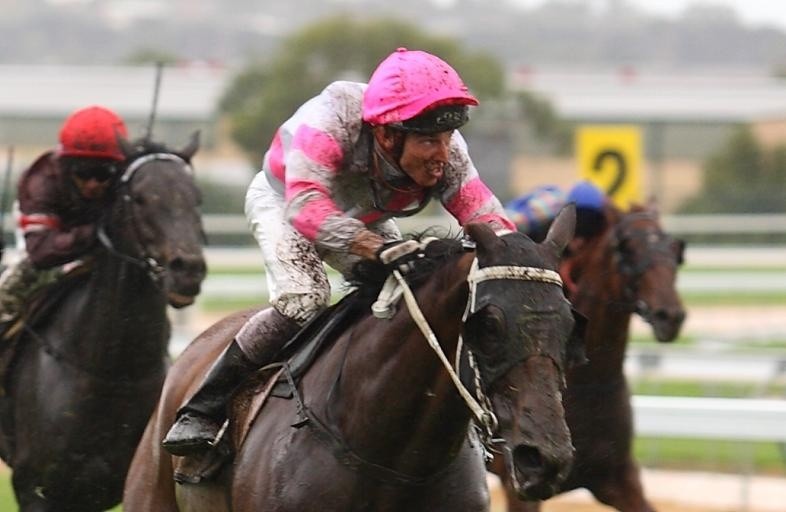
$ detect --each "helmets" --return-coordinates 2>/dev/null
[59,105,128,161]
[362,47,480,133]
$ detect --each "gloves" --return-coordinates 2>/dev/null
[375,240,427,275]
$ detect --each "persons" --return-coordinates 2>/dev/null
[0,105,130,347]
[503,179,610,241]
[161,46,517,458]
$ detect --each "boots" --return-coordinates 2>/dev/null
[162,338,261,456]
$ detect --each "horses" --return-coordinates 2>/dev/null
[119,198,580,512]
[0,124,209,512]
[481,190,688,512]
